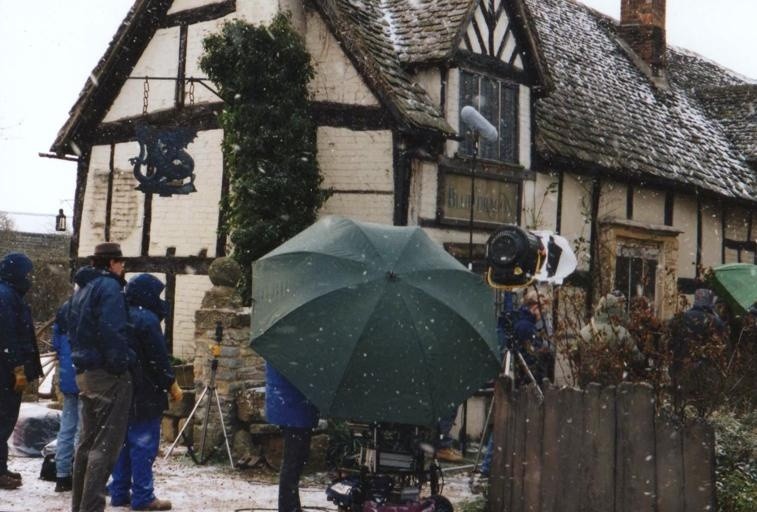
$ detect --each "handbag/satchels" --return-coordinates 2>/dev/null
[41,454,58,480]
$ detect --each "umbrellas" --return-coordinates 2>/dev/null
[246,215,505,431]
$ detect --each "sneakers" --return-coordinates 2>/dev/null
[0,471,22,489]
[54,477,74,493]
[121,494,171,509]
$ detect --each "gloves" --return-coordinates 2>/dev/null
[169,382,183,403]
[12,366,29,393]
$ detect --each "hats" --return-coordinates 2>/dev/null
[89,243,129,261]
[693,290,713,307]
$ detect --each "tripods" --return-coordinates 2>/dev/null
[158,323,250,470]
[468,349,545,484]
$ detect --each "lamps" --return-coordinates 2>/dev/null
[56,198,75,231]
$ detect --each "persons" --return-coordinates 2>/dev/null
[432,406,461,463]
[50,264,102,494]
[65,242,137,511]
[509,291,554,383]
[0,252,44,489]
[262,359,323,512]
[108,273,186,511]
[572,288,755,392]
